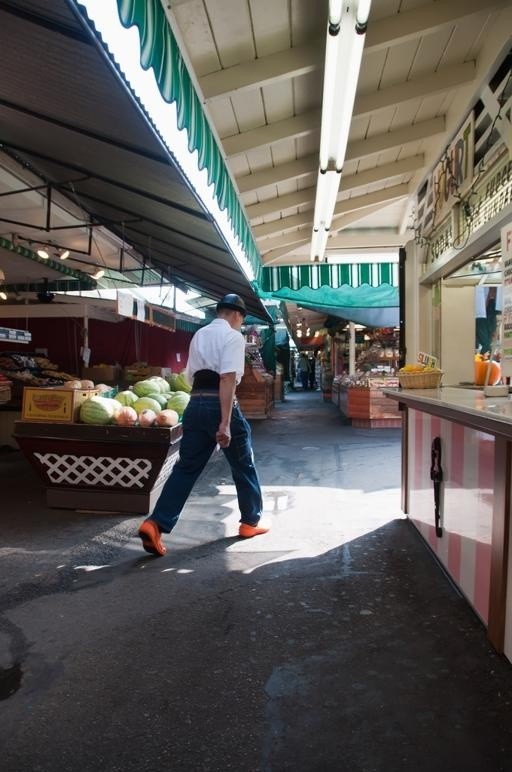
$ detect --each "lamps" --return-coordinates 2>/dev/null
[312,229,328,262]
[327,0,373,24]
[81,266,105,279]
[314,172,342,230]
[55,246,70,260]
[318,23,367,172]
[36,241,51,259]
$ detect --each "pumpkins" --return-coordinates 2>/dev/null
[63,380,112,392]
[475,360,500,386]
[115,407,178,427]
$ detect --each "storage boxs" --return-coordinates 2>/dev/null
[21,385,99,423]
[123,366,171,384]
[81,366,120,386]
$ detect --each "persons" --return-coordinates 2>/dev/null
[139,293,269,556]
[298,353,316,389]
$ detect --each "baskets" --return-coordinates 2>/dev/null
[396,371,445,388]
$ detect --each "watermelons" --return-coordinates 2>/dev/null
[115,373,192,416]
[107,398,122,411]
[80,396,113,425]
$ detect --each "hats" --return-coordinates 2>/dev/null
[217,293,247,316]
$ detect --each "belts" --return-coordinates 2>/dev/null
[191,392,219,397]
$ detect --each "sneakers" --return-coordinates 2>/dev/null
[239,524,270,537]
[139,521,166,556]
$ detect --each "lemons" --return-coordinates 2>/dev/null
[399,363,440,373]
